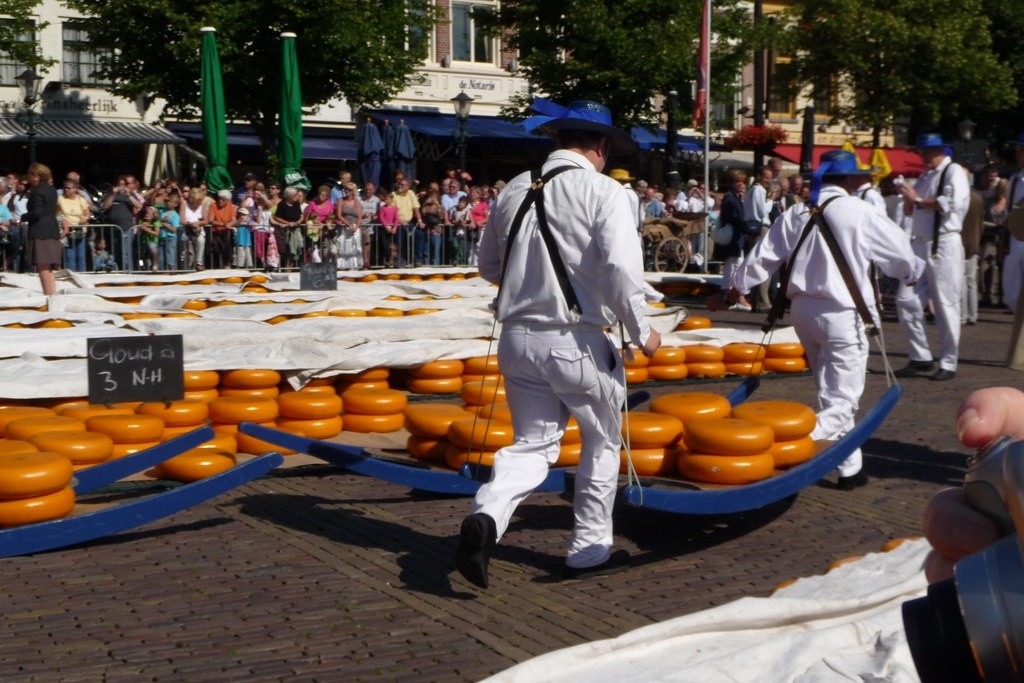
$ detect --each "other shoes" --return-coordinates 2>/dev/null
[839,469,868,490]
[563,549,631,579]
[454,513,496,590]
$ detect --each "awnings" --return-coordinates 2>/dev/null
[177,129,358,161]
[374,110,730,151]
[0,118,183,144]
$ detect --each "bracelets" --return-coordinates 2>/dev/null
[187,222,189,224]
[915,197,921,205]
[418,220,422,221]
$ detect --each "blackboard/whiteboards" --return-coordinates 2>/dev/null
[87,334,185,404]
[300,263,337,290]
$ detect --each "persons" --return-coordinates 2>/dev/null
[378,170,505,270]
[707,143,1024,325]
[921,387,1024,583]
[146,172,381,271]
[21,164,64,295]
[732,150,925,489]
[893,134,970,378]
[0,172,163,272]
[455,101,661,589]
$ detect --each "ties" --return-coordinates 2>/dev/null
[778,199,783,212]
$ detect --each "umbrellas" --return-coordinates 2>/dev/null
[358,123,415,191]
[799,106,814,180]
[841,141,891,185]
[280,32,311,192]
[200,27,233,193]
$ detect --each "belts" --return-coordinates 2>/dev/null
[914,231,960,240]
[501,320,602,332]
[793,295,837,307]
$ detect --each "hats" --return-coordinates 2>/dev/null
[688,178,698,188]
[244,172,257,180]
[905,133,954,157]
[238,208,249,215]
[609,169,635,181]
[518,96,634,156]
[807,151,871,205]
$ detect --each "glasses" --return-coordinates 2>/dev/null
[448,185,457,189]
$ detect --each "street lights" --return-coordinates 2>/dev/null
[450,92,474,173]
[13,70,45,167]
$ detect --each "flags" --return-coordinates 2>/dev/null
[693,1,707,126]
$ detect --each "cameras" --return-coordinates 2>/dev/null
[899,433,1024,683]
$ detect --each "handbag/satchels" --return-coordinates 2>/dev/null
[1006,197,1024,242]
[710,221,733,246]
[422,215,440,225]
[745,221,761,235]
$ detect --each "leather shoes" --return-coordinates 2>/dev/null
[930,368,956,380]
[894,360,934,378]
